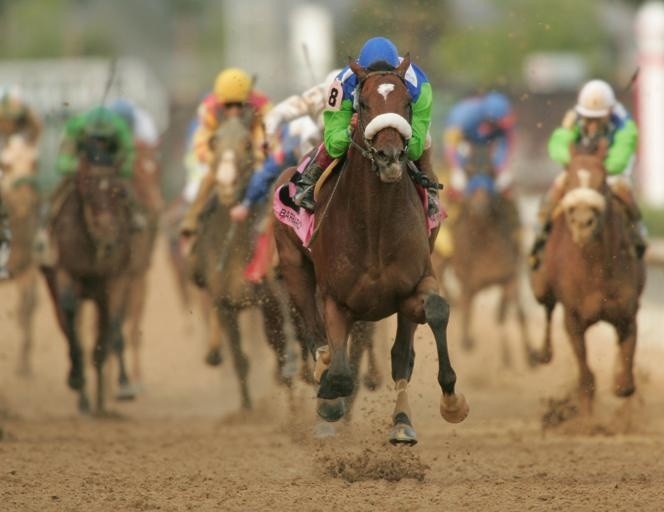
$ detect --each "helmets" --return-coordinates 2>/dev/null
[113,100,134,127]
[0,95,27,119]
[84,106,114,137]
[483,93,510,124]
[216,68,253,103]
[357,36,399,73]
[574,80,616,119]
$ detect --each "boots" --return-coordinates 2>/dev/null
[295,143,338,210]
[636,219,647,259]
[529,220,552,269]
[414,147,439,214]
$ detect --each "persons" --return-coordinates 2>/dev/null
[293,36,441,218]
[260,68,344,157]
[34,105,148,251]
[0,91,44,194]
[230,115,320,222]
[109,99,164,150]
[443,92,523,201]
[181,68,275,236]
[526,79,648,270]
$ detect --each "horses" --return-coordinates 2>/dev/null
[1,54,533,443]
[531,139,647,428]
